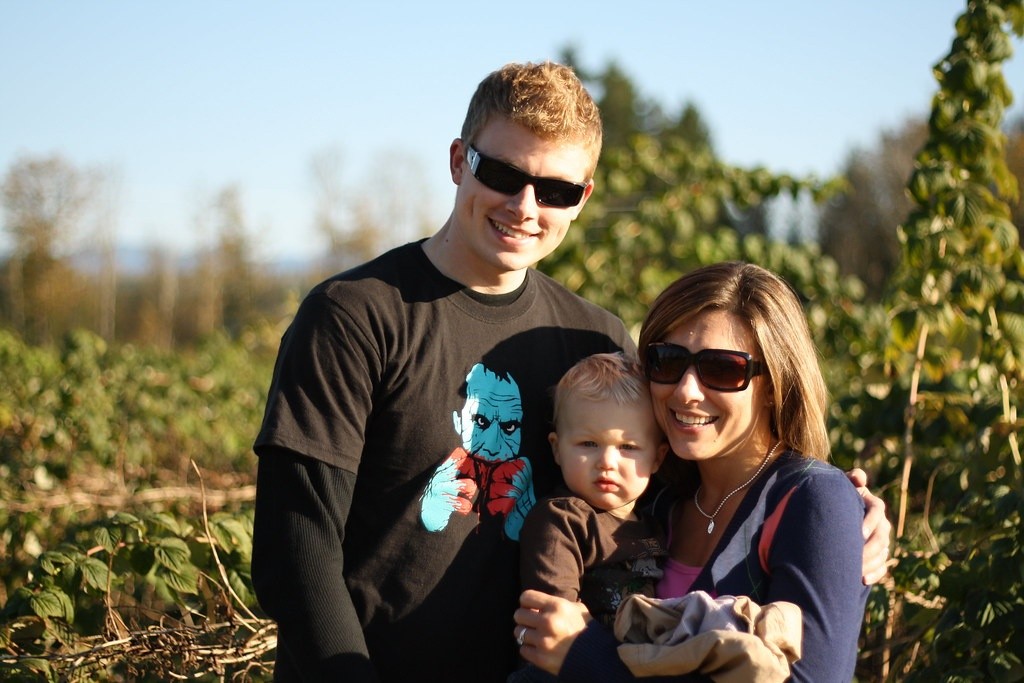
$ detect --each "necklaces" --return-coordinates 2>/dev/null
[694,436,785,535]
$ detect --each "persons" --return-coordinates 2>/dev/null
[251,57,893,682]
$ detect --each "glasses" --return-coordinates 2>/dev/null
[642,342,771,392]
[465,143,592,209]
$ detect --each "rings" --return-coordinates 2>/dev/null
[517,628,529,645]
